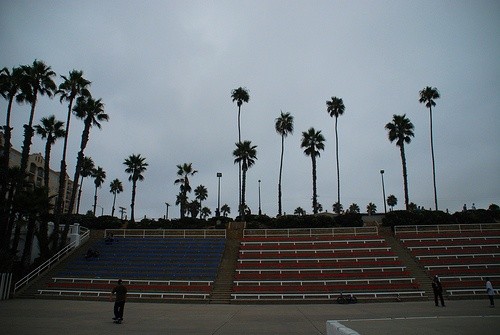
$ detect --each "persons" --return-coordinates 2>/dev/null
[431,275,445,306]
[109,279,127,321]
[472,203,475,209]
[463,204,467,210]
[485,277,495,308]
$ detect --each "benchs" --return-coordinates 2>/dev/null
[231,230,500,300]
[38,237,227,299]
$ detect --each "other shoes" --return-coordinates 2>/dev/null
[112,317,121,321]
[113,321,121,323]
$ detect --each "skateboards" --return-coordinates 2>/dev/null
[112,318,123,324]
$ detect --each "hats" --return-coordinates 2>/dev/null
[117,280,123,282]
